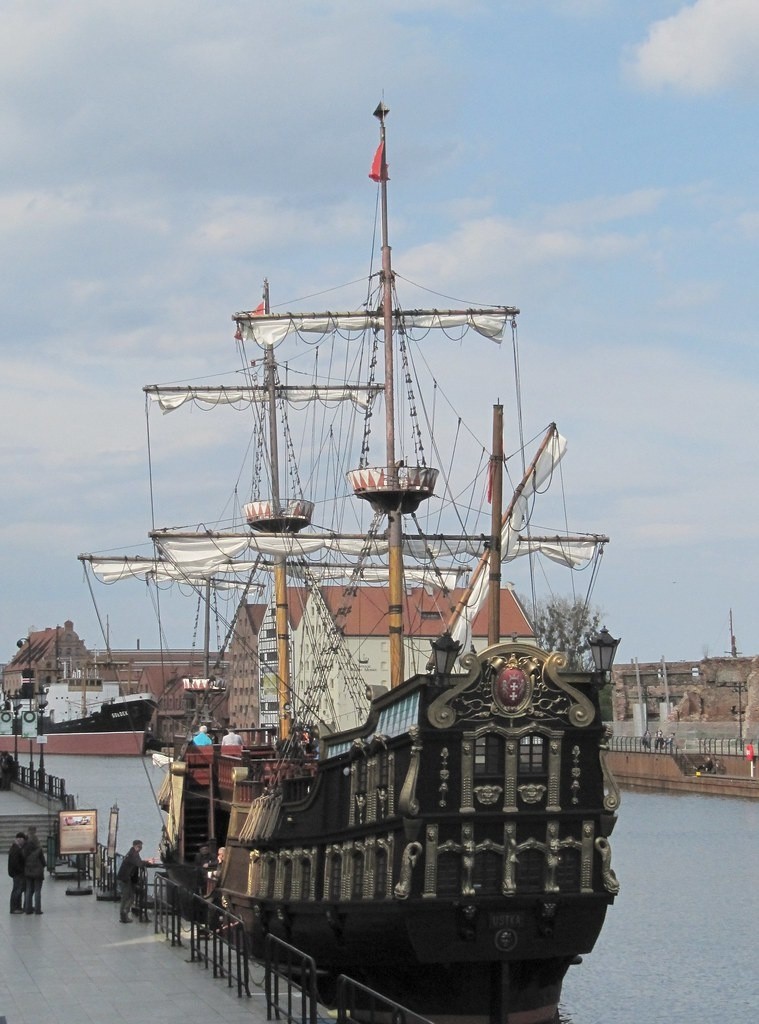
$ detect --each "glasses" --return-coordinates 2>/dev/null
[137,846,142,849]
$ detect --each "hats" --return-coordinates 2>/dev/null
[16,832,27,840]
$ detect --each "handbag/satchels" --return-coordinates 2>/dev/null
[131,868,139,883]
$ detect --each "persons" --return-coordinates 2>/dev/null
[221,726,244,746]
[0,750,15,790]
[695,756,712,772]
[191,725,212,747]
[642,730,676,752]
[23,825,37,912]
[8,832,26,914]
[192,845,225,875]
[23,836,47,914]
[117,840,155,923]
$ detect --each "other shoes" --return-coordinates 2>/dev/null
[26,911,33,914]
[10,908,24,914]
[119,917,133,922]
[121,911,127,923]
[36,910,43,914]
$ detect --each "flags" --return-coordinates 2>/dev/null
[234,302,265,340]
[368,140,384,183]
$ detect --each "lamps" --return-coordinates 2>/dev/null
[588,626,622,688]
[429,633,462,687]
